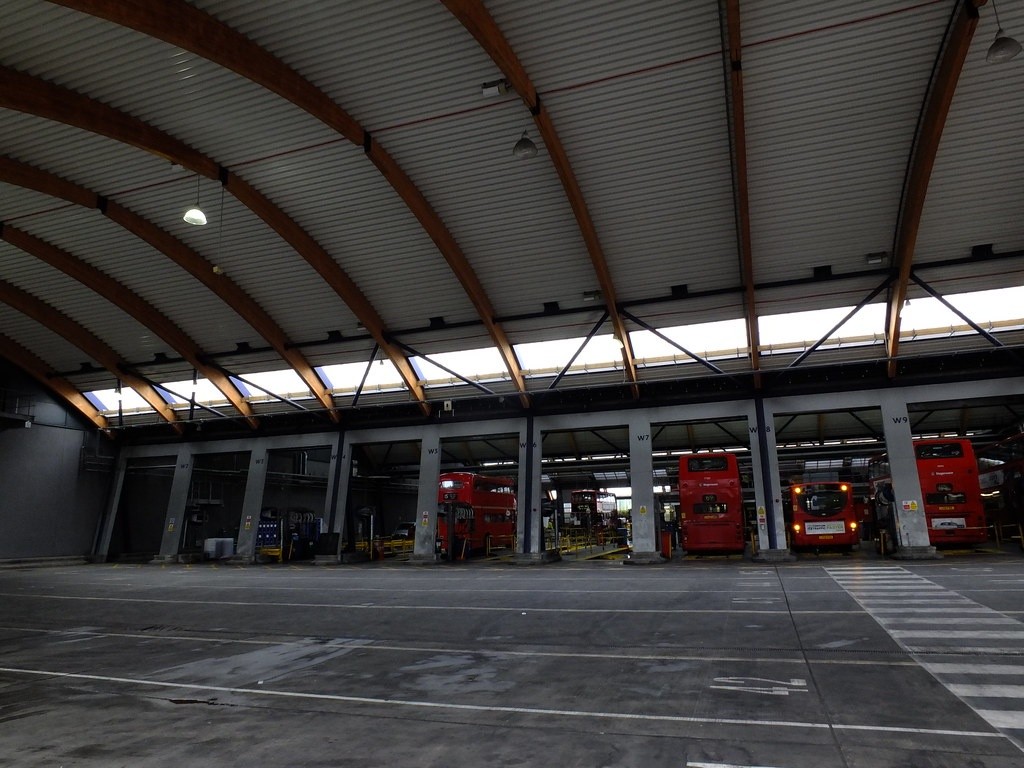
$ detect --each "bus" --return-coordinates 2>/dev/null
[437,472,517,554]
[571,490,617,516]
[678,452,745,551]
[867,438,987,543]
[790,482,860,552]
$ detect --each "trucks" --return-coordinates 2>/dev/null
[256,509,323,563]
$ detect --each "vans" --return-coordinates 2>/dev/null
[395,522,416,538]
